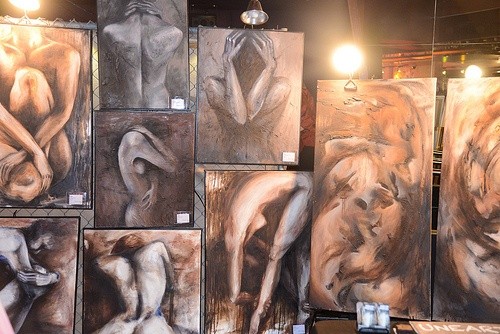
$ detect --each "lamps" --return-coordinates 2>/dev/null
[239,0,270,29]
